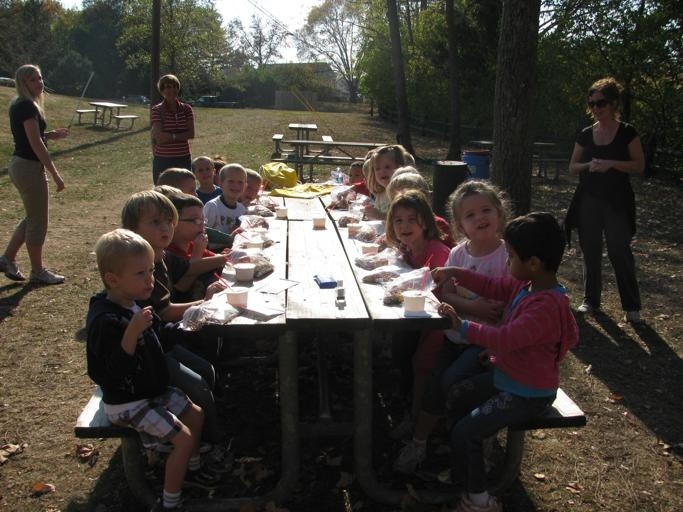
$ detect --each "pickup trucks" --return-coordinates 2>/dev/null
[192,96,240,110]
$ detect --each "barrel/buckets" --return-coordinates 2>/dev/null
[432,159,469,219]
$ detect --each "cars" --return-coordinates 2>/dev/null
[0,78,14,87]
[177,96,194,106]
[119,94,152,105]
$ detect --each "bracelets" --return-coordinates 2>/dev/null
[172,133,176,143]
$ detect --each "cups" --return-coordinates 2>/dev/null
[275,207,287,219]
[401,290,427,313]
[312,218,325,230]
[245,240,263,250]
[223,286,247,308]
[359,243,379,255]
[346,223,362,239]
[232,263,256,282]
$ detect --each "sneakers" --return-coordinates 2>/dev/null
[182,466,224,490]
[148,497,193,512]
[200,440,234,472]
[387,420,415,447]
[29,268,65,285]
[622,311,639,323]
[0,254,26,281]
[449,492,505,512]
[393,436,428,473]
[577,300,595,313]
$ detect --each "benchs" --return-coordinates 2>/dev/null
[322,134,334,155]
[113,115,140,131]
[473,140,570,182]
[76,109,101,124]
[273,158,366,182]
[453,388,585,500]
[73,384,204,512]
[273,134,283,157]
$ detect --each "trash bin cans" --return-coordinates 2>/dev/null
[432,160,467,213]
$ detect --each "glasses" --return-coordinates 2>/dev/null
[176,216,208,225]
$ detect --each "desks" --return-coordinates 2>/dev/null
[282,139,388,161]
[288,123,317,154]
[89,101,128,127]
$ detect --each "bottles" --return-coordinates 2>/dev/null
[334,167,343,186]
[335,280,345,304]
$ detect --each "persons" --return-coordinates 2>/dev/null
[564,78,646,325]
[0,63,69,285]
[151,73,194,187]
[76,156,262,512]
[337,144,579,511]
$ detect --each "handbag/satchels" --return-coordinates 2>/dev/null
[149,103,166,151]
[259,160,298,192]
